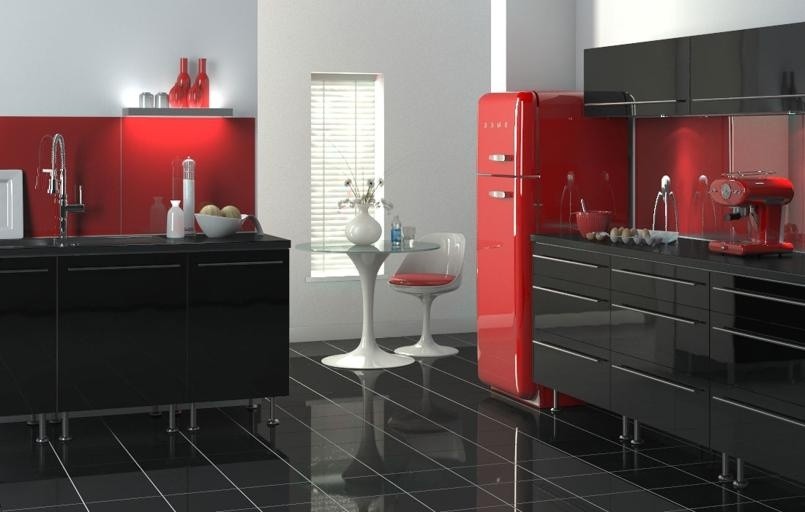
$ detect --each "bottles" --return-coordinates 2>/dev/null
[165,200,185,239]
[177,58,190,90]
[168,82,186,109]
[391,213,401,248]
[182,156,196,237]
[149,197,166,234]
[196,58,209,108]
[187,80,203,108]
[171,155,182,200]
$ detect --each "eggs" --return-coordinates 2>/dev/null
[609,226,652,237]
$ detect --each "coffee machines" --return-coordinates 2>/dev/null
[707,171,796,257]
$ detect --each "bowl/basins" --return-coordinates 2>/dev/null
[576,211,614,238]
[193,213,246,238]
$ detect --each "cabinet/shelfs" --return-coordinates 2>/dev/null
[185,234,291,432]
[529,231,633,442]
[53,236,187,442]
[582,34,691,118]
[711,249,805,495]
[612,237,733,485]
[0,235,58,445]
[690,20,805,118]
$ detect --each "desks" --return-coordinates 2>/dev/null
[291,237,452,374]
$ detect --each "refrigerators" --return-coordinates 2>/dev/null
[473,90,629,409]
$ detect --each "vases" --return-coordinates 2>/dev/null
[192,57,211,108]
[344,203,385,246]
[174,53,193,108]
[165,198,187,239]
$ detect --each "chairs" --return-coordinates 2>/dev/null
[385,229,468,360]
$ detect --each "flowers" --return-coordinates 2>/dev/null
[335,174,391,210]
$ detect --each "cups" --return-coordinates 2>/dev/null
[138,93,154,109]
[402,225,416,249]
[154,92,169,109]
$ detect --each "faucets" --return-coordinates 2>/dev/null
[46,132,85,241]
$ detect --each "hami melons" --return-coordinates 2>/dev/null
[221,205,241,219]
[200,205,221,217]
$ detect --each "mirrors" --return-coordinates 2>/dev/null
[629,113,805,253]
